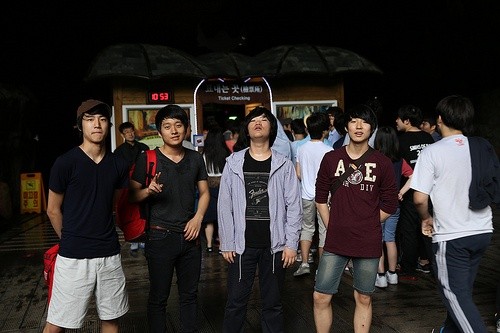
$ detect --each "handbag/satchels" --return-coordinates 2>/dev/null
[207,175,221,188]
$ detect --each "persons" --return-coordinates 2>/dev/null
[373,126,413,288]
[217,106,303,333]
[183,123,248,254]
[395,104,434,274]
[409,95,495,333]
[420,113,443,142]
[113,121,150,251]
[129,105,210,333]
[42,100,130,333]
[293,111,336,276]
[273,106,378,271]
[313,103,399,333]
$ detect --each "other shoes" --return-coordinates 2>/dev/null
[206,246,213,252]
[218,250,222,254]
[399,274,416,280]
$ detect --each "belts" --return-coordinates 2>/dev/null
[150,226,168,231]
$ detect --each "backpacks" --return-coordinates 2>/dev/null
[115,149,157,239]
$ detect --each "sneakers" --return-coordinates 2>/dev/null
[293,263,310,276]
[375,271,398,287]
[416,263,431,273]
[130,242,145,251]
[297,250,314,263]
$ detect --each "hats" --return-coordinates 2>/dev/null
[77,99,112,120]
